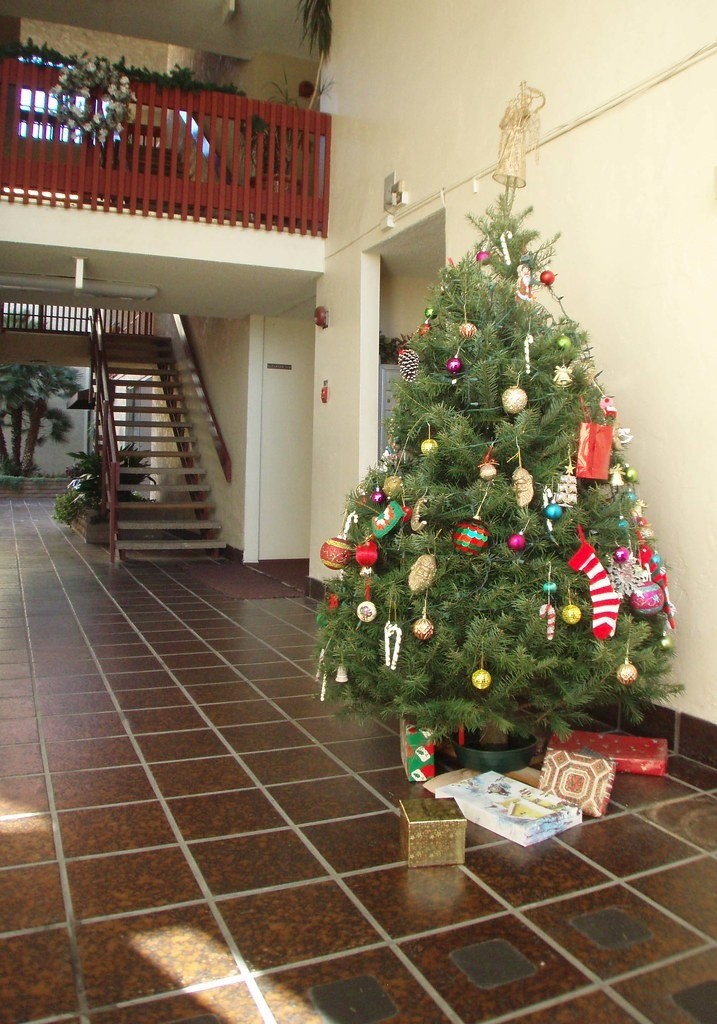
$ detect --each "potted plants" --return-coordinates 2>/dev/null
[312,75,687,776]
[50,442,165,545]
[241,61,337,228]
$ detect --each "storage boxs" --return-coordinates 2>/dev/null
[398,796,468,868]
[398,712,435,782]
[422,729,670,848]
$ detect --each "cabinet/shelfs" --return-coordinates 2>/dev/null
[378,363,400,468]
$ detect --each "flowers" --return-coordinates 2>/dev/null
[52,55,138,144]
